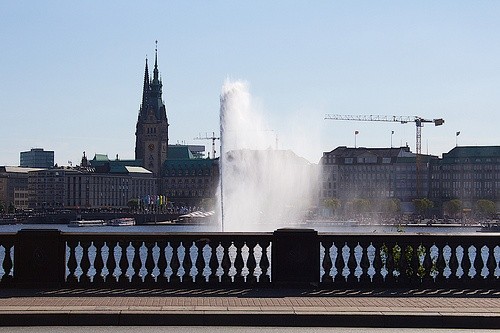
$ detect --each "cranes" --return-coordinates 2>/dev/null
[324,114,444,196]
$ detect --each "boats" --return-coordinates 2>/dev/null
[474,222,500,232]
[108,218,136,226]
[69,219,106,227]
